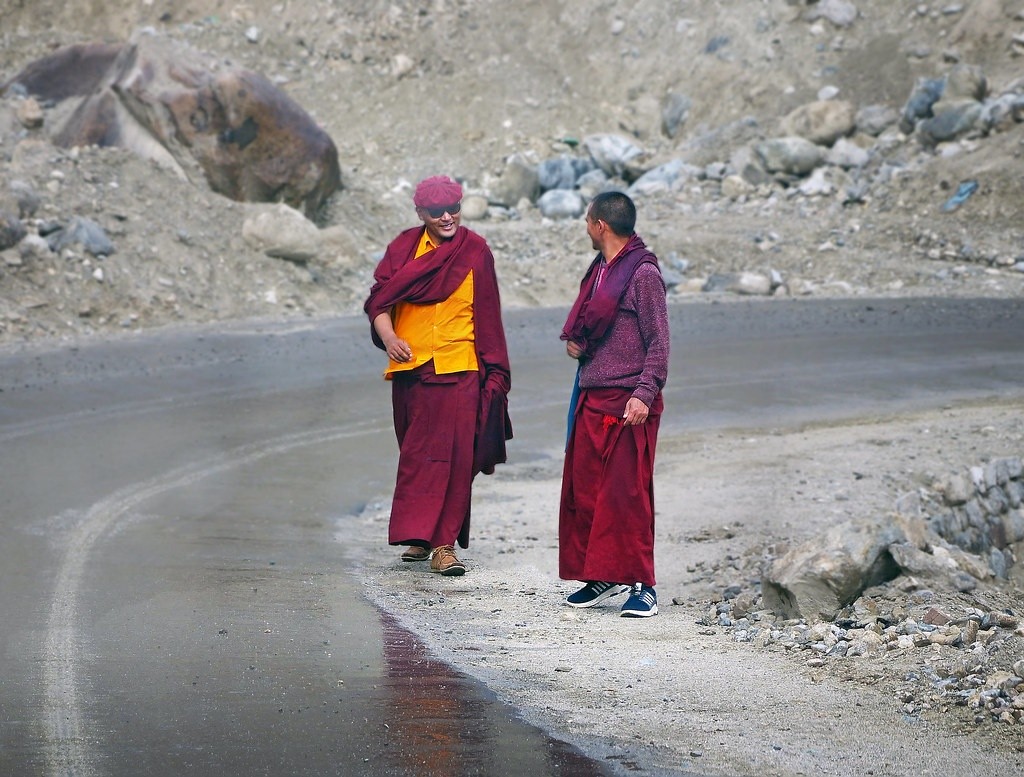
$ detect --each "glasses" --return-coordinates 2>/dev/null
[428,203,461,218]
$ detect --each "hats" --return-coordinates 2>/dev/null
[414,176,462,208]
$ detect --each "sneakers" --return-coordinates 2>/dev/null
[566,580,630,608]
[620,584,658,617]
[400,546,431,561]
[430,545,465,576]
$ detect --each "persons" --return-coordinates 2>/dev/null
[558,191,671,620]
[363,176,513,574]
